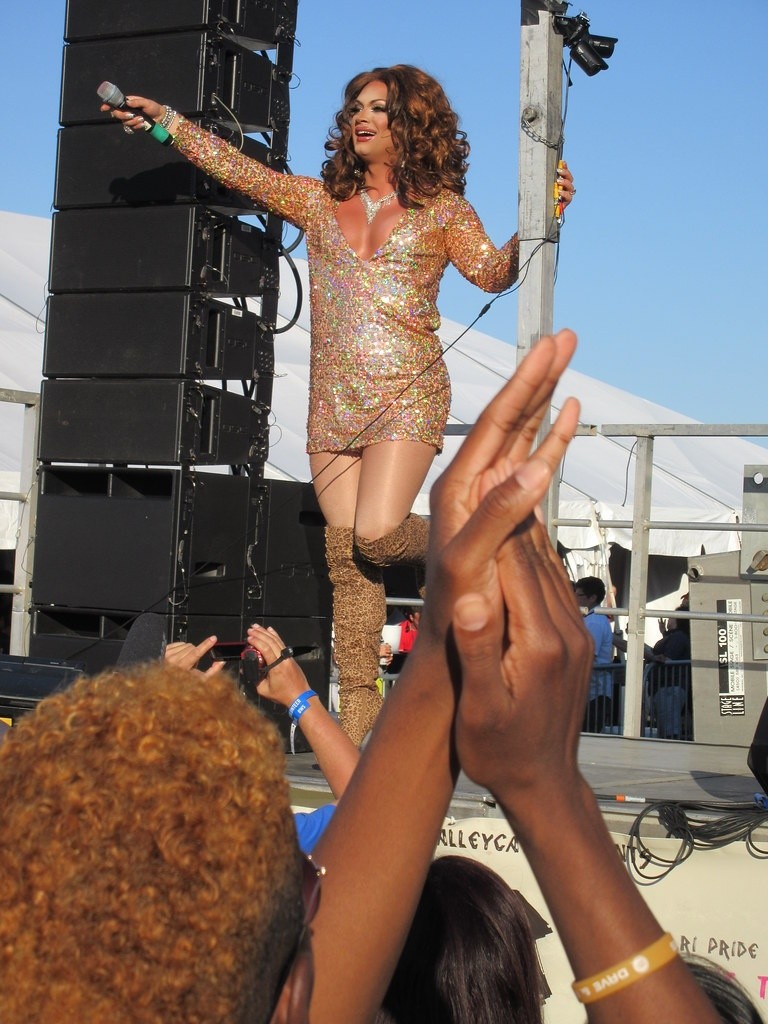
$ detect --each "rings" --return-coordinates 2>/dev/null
[123,125,134,135]
[570,188,576,195]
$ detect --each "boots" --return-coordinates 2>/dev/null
[352,512,433,600]
[310,525,388,773]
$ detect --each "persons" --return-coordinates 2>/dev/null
[100,64,575,770]
[0,328,768,1024]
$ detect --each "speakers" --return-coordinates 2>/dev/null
[28,0,336,715]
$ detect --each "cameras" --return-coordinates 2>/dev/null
[209,642,266,672]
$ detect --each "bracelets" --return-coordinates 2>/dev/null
[569,930,678,1003]
[160,104,177,131]
[286,685,319,759]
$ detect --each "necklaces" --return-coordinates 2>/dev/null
[354,168,413,225]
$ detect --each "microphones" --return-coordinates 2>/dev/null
[96,81,175,147]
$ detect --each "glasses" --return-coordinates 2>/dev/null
[574,591,586,598]
